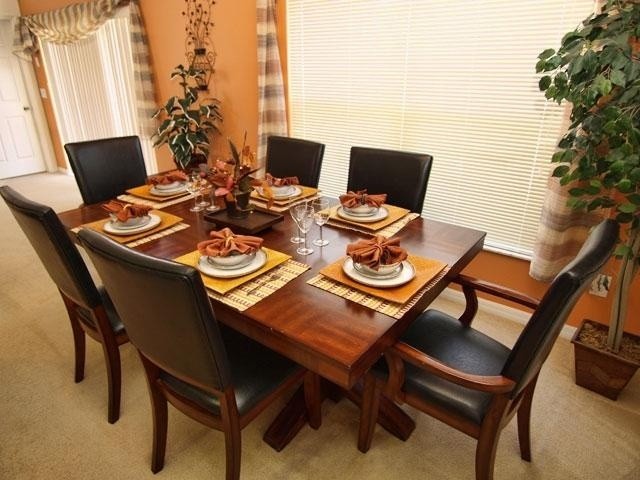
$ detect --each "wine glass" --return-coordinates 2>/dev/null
[287,194,331,255]
[186,173,219,212]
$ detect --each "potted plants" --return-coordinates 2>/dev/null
[536,0,640,401]
[149,65,225,174]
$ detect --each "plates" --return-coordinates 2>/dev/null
[334,205,389,223]
[101,214,161,237]
[150,182,187,195]
[255,186,302,201]
[198,248,270,277]
[340,254,417,289]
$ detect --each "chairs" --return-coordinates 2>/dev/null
[357,218,621,480]
[63,136,150,205]
[347,147,433,218]
[265,136,325,189]
[0,186,131,423]
[77,230,323,479]
